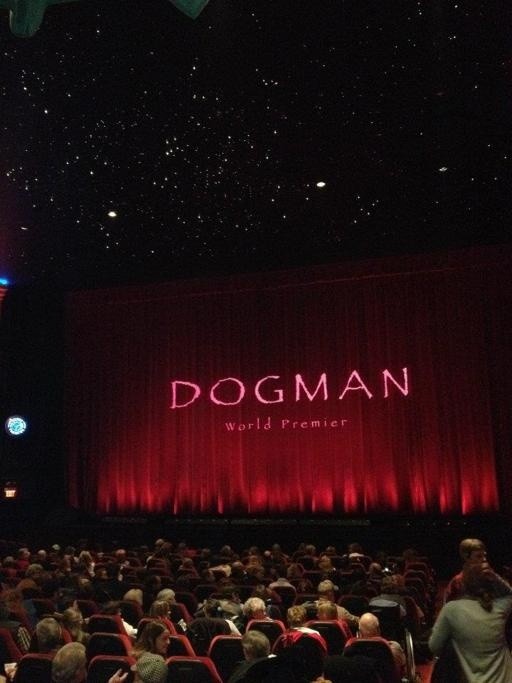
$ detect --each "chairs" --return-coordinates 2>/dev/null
[0,554,431,683]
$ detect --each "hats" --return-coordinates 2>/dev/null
[317,580,339,593]
